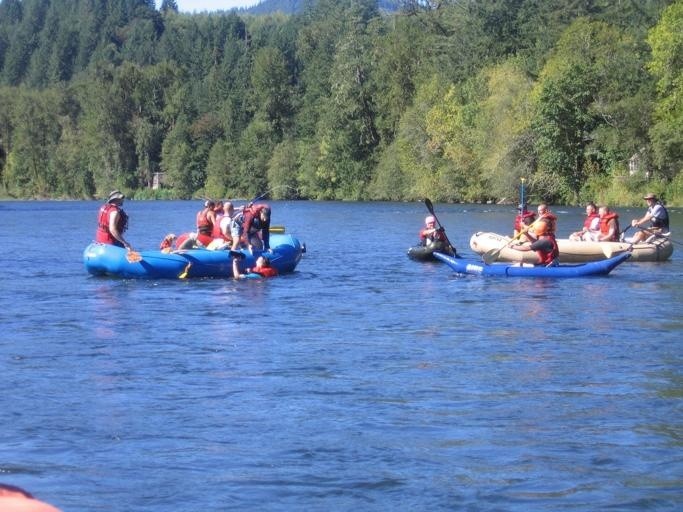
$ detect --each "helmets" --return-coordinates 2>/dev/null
[203,200,216,208]
[424,215,436,225]
[532,221,548,237]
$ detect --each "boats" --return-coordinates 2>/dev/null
[432,244,634,277]
[407,238,449,263]
[408,243,455,262]
[433,252,631,277]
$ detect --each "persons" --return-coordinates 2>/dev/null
[629,193,670,245]
[419,216,447,247]
[517,204,558,244]
[232,256,277,280]
[188,200,217,248]
[512,205,536,245]
[213,200,224,216]
[582,206,619,242]
[230,204,273,256]
[569,204,600,242]
[506,221,559,266]
[207,202,234,250]
[96,189,132,252]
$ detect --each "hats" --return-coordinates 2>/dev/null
[643,191,659,202]
[106,188,127,203]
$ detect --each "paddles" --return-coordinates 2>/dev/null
[482,211,549,264]
[425,198,461,258]
[269,226,285,234]
[126,245,143,263]
